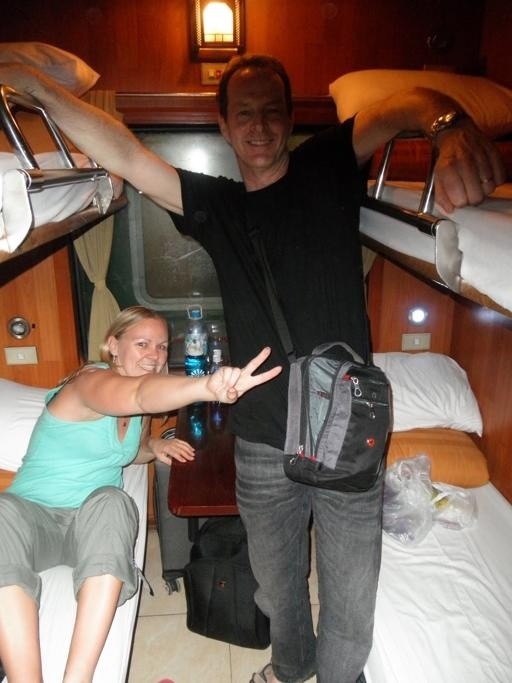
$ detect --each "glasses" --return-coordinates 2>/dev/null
[191,0,241,48]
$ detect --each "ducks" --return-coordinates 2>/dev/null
[153,457,211,596]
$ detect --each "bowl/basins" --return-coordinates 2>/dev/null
[121,418,127,427]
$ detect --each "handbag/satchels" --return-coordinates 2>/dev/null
[428,106,464,136]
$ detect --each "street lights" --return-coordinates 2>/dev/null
[284,337,396,497]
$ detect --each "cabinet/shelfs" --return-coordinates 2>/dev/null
[207,323,227,372]
[188,402,208,447]
[180,302,209,376]
[211,400,223,434]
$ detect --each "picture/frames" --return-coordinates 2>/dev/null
[168,402,240,543]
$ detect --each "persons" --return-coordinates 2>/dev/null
[0,307,284,683]
[0,52,507,683]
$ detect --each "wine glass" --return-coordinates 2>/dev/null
[480,174,494,186]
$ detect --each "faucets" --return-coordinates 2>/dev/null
[250,662,298,683]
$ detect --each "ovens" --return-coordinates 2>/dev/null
[0,86,150,683]
[358,128,512,682]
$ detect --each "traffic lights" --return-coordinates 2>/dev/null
[372,351,484,437]
[0,41,101,101]
[329,67,512,127]
[0,378,53,473]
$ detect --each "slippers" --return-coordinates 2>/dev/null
[183,518,274,650]
